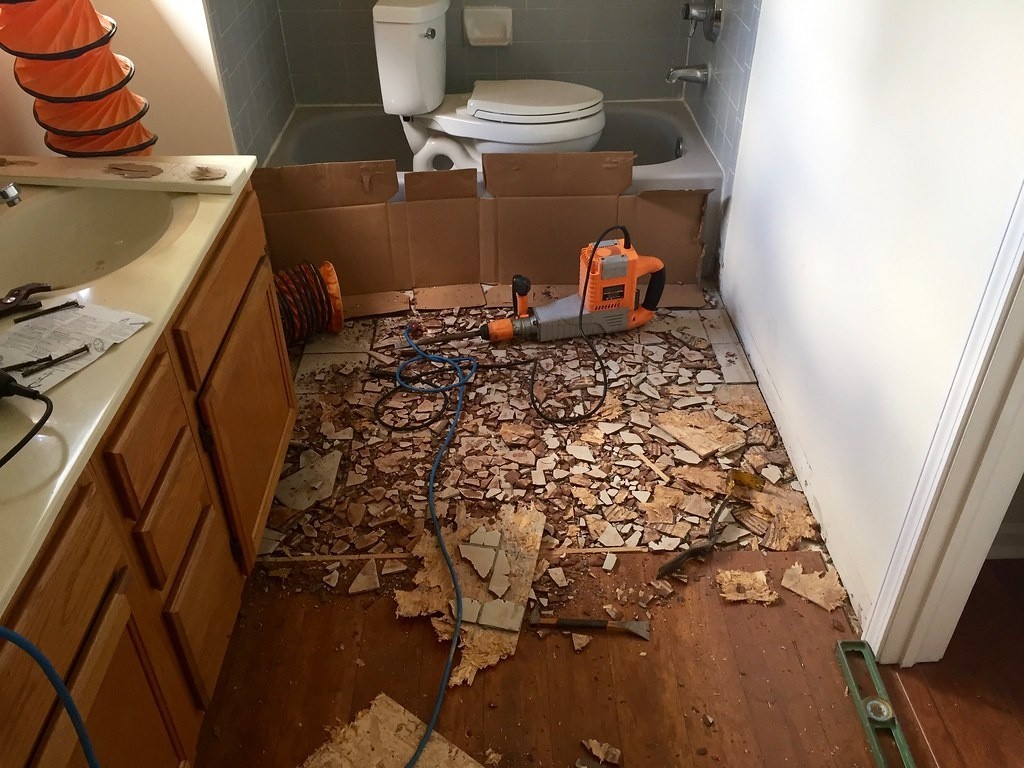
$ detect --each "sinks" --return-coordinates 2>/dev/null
[0,185,200,306]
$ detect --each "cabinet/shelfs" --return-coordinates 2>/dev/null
[0,178,299,768]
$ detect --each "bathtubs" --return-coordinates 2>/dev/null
[263,100,723,284]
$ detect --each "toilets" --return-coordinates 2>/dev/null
[372,0,605,183]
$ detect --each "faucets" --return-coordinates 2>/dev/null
[1,176,22,206]
[666,65,708,87]
